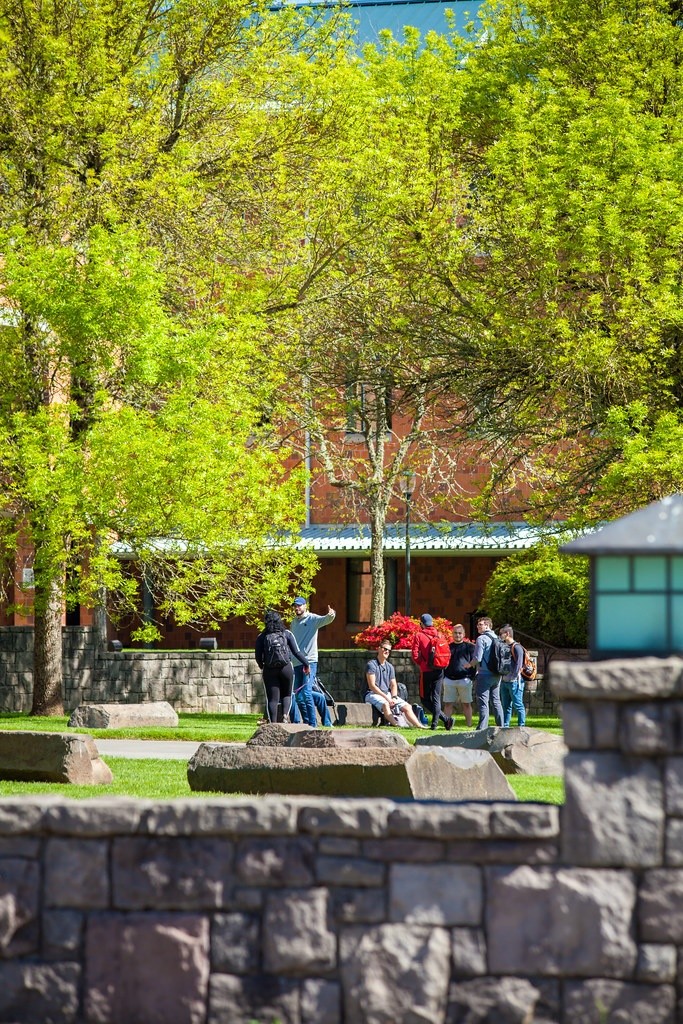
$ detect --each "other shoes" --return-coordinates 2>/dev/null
[444,718,454,730]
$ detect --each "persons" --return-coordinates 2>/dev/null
[412,614,526,731]
[365,639,431,729]
[255,597,335,729]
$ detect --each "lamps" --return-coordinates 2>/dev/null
[556,490,683,658]
[108,639,123,652]
[199,638,218,652]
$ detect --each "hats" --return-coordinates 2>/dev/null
[420,613,433,627]
[291,597,306,607]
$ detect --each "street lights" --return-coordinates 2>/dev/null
[398,469,418,617]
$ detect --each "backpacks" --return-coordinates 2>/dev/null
[483,632,512,676]
[425,631,451,669]
[412,703,424,724]
[312,676,334,706]
[512,642,536,680]
[263,628,290,666]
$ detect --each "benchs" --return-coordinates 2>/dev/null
[315,702,385,726]
[67,701,178,728]
[186,742,515,801]
[0,729,114,785]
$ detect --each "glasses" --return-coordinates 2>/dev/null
[420,622,423,625]
[454,631,463,634]
[381,647,391,653]
